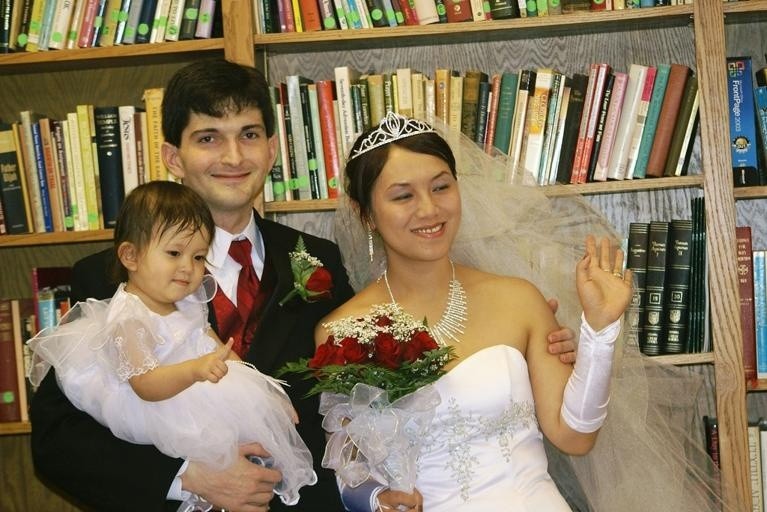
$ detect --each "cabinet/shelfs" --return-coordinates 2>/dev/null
[0,0,767,512]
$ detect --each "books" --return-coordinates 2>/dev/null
[1,87,183,235]
[0,259,73,426]
[1,0,217,54]
[263,62,700,202]
[733,224,767,382]
[626,206,711,358]
[699,412,767,511]
[728,52,767,188]
[253,1,691,35]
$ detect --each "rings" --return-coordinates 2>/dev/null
[601,267,613,273]
[614,271,625,279]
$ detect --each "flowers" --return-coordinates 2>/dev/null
[277,231,334,308]
[272,300,458,406]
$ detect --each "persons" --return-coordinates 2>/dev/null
[29,62,361,512]
[314,112,634,510]
[110,181,298,462]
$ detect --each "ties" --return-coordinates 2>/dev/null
[228,238,261,322]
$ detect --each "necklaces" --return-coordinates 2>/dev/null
[380,256,469,355]
